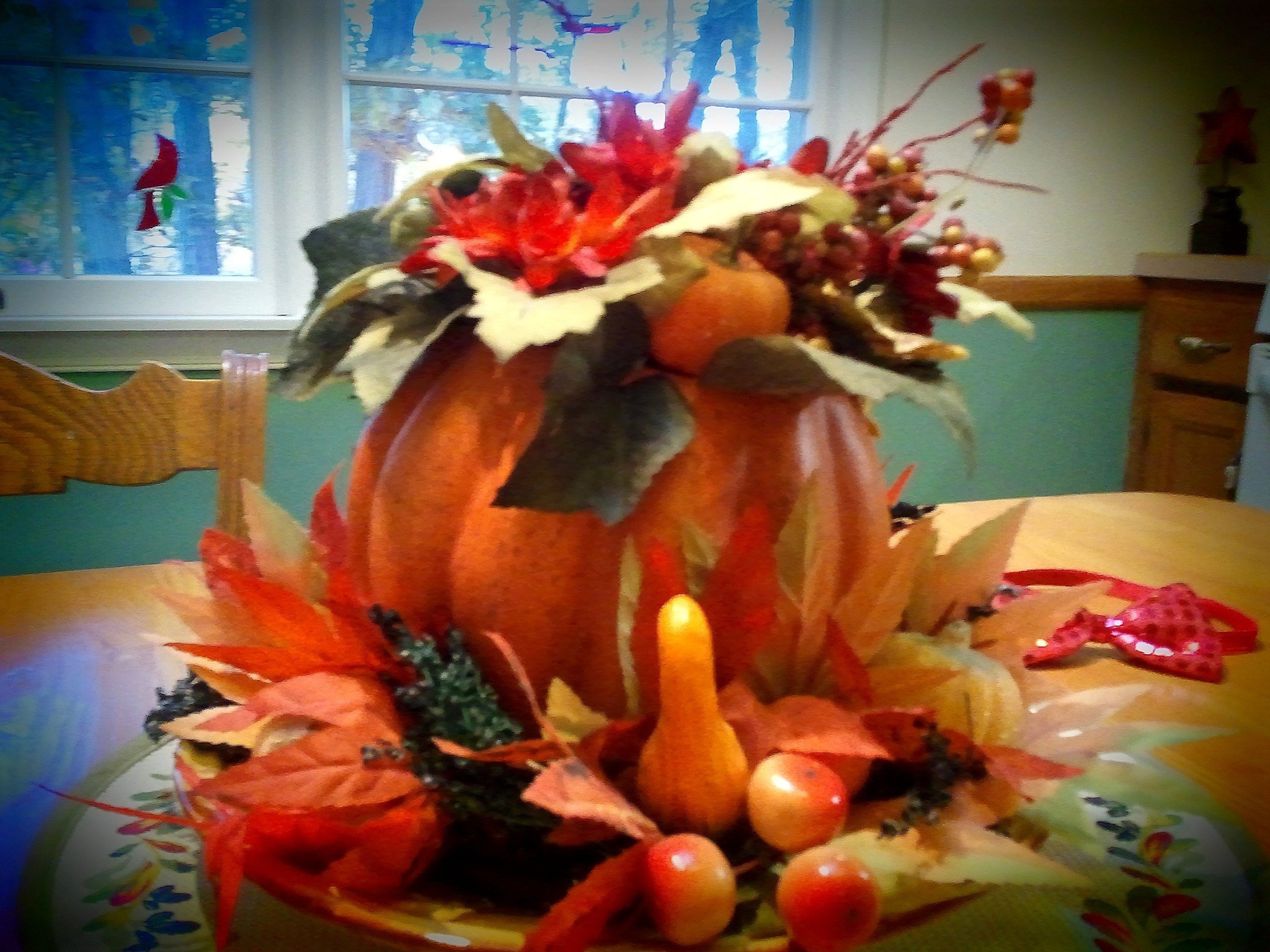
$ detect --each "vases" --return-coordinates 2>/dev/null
[1188,189,1251,255]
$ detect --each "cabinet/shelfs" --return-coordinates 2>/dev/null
[1122,282,1260,502]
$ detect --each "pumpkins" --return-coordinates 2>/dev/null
[645,213,791,377]
[350,316,896,723]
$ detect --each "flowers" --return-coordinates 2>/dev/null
[1193,87,1260,187]
[35,42,1091,952]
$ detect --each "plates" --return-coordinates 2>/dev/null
[170,739,657,952]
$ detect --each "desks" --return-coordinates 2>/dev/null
[0,494,1270,952]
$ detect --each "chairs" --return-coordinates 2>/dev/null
[0,350,270,546]
